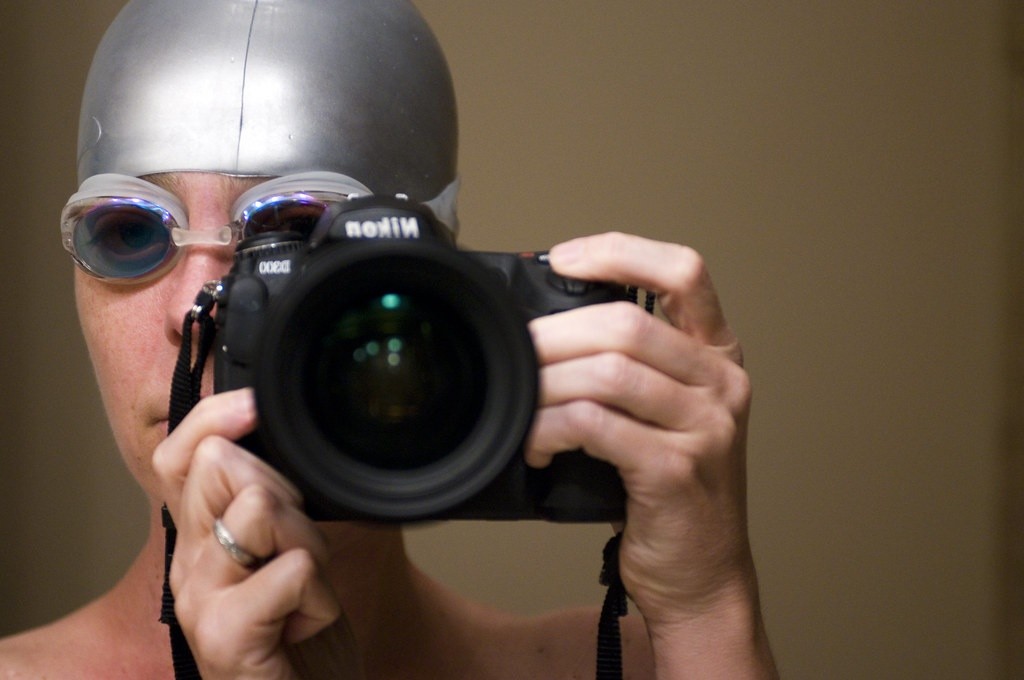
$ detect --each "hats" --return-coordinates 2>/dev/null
[74,0,461,203]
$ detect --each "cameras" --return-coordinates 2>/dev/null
[210,195,636,525]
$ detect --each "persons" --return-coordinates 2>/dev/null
[0,0,779,680]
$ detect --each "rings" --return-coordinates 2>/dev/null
[212,518,265,572]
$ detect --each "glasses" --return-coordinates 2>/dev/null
[57,174,460,285]
